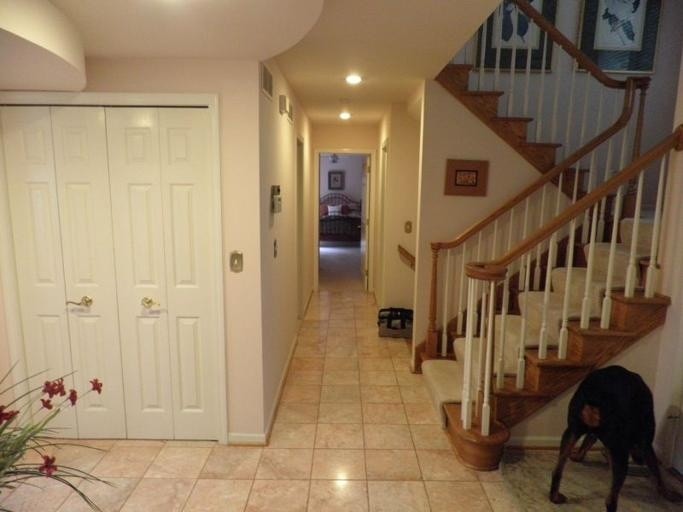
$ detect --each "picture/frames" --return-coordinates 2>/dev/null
[472,1,663,74]
[328,171,344,190]
[444,158,489,197]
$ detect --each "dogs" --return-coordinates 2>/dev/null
[548,364,683,512]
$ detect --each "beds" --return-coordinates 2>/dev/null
[320,192,361,241]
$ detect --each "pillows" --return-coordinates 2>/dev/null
[320,204,350,216]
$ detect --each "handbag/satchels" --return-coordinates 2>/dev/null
[376,307,413,338]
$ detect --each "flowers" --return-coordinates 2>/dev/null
[0,357,118,511]
